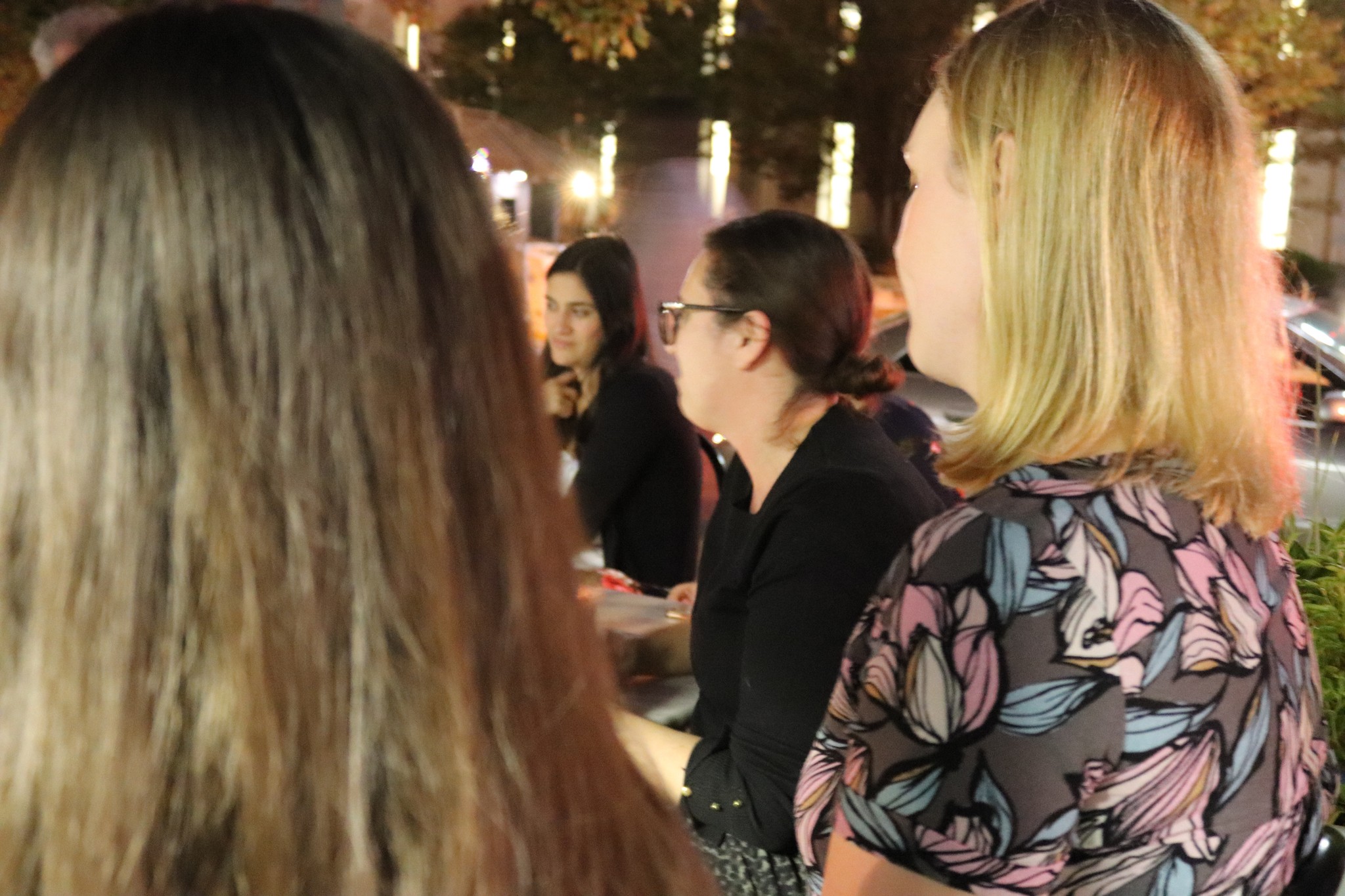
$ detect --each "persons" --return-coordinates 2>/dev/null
[537,237,701,598]
[602,211,947,896]
[791,0,1325,896]
[0,1,720,896]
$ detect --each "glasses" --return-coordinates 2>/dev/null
[656,300,748,348]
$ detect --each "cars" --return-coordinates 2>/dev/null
[863,290,1345,538]
